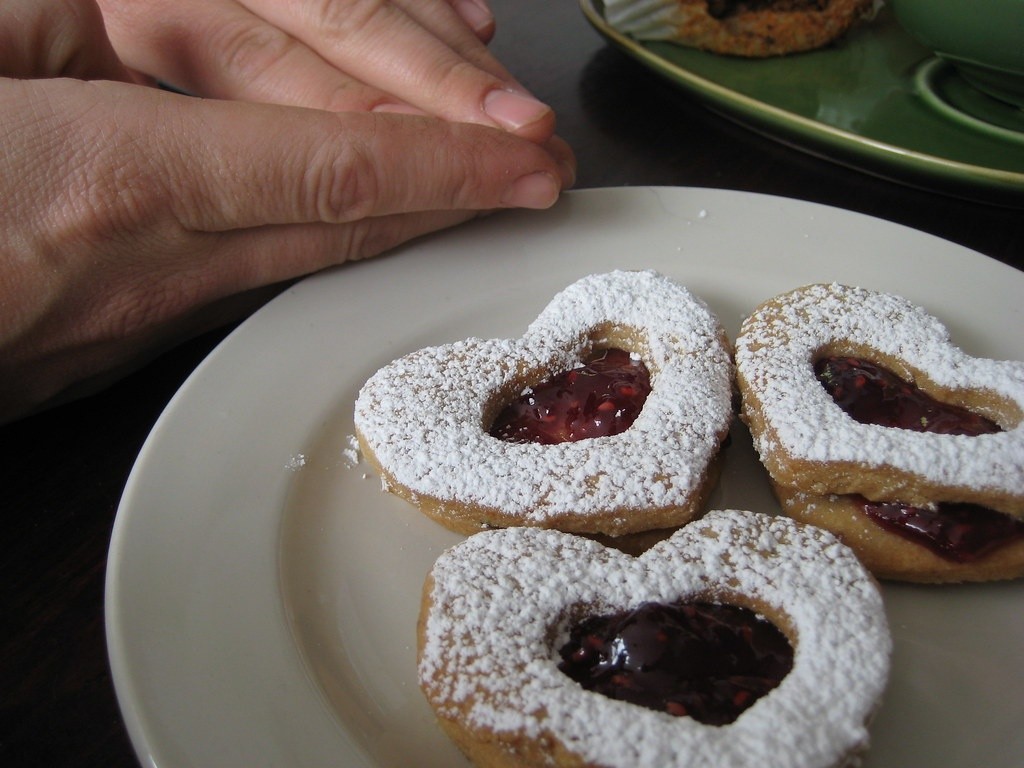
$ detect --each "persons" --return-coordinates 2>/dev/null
[1,1,579,431]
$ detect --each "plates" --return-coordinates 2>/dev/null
[578,0,1024,202]
[106,184,1024,768]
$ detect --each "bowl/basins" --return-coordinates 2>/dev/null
[888,0,1024,109]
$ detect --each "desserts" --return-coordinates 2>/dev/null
[354,267,741,543]
[730,283,1024,584]
[415,507,894,766]
